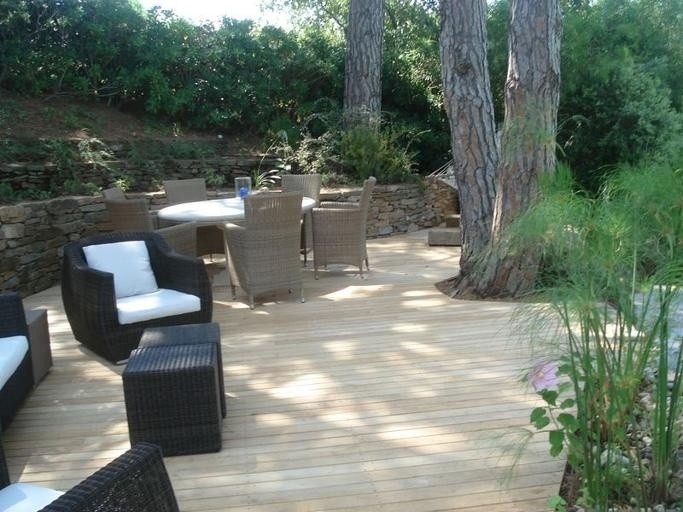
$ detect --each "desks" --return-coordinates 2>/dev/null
[157,196,317,267]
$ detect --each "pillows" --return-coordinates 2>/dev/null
[82,240,159,299]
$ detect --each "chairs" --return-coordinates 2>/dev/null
[105,199,198,256]
[223,191,306,309]
[102,187,160,230]
[61,232,213,366]
[162,178,225,261]
[312,176,377,279]
[0,442,180,512]
[281,174,322,266]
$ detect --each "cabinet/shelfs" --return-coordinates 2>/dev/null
[26,309,53,384]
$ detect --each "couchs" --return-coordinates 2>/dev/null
[0,292,36,490]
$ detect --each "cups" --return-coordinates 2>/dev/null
[235,177,251,207]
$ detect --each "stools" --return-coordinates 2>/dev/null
[138,323,226,419]
[122,344,224,458]
